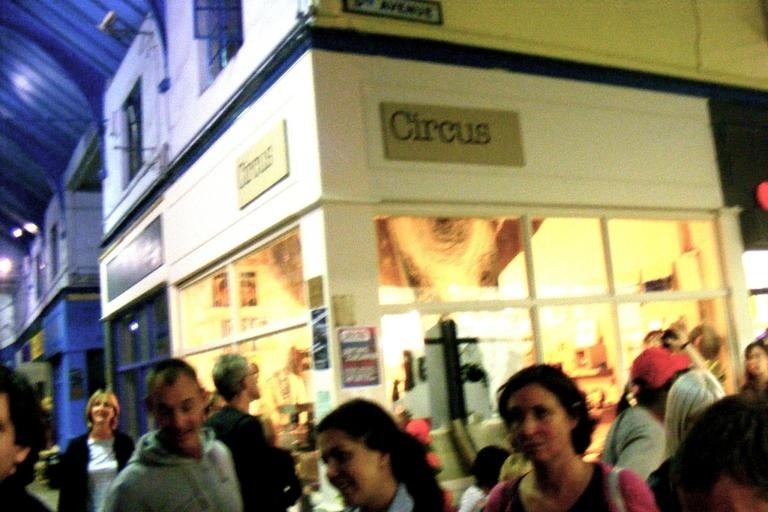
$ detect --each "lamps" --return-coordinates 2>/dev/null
[94,10,155,40]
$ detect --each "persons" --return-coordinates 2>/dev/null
[94,356,245,512]
[202,353,305,510]
[313,395,456,511]
[46,386,135,512]
[1,361,55,512]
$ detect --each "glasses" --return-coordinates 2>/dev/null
[242,363,261,382]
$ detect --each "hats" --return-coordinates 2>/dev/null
[630,345,693,391]
